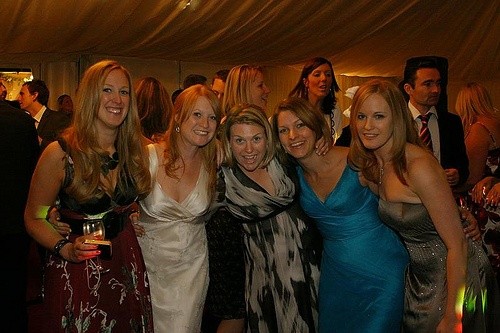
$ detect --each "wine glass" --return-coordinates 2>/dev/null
[83,220,110,274]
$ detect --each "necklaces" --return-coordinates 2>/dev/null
[240,166,262,173]
[378,159,389,185]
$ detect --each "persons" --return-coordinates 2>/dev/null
[23,59,156,333]
[346,80,493,333]
[274,101,482,333]
[403,58,470,207]
[0,99,39,333]
[48,83,221,333]
[285,56,344,145]
[203,65,272,333]
[19,79,68,155]
[454,82,500,248]
[129,102,334,333]
[55,69,229,143]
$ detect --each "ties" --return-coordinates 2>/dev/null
[417,112,434,154]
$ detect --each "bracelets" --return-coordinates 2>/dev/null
[54,239,71,256]
